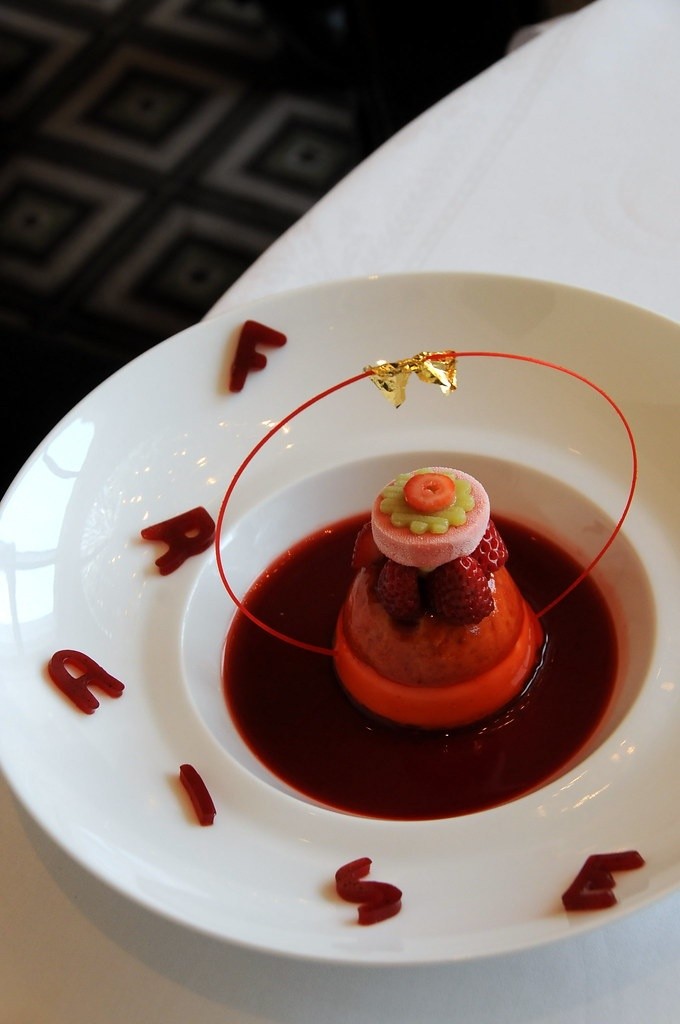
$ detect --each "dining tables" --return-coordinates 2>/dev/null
[0,1,680,1023]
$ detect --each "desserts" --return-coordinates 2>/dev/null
[332,463,544,727]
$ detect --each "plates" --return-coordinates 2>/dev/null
[0,275,679,963]
